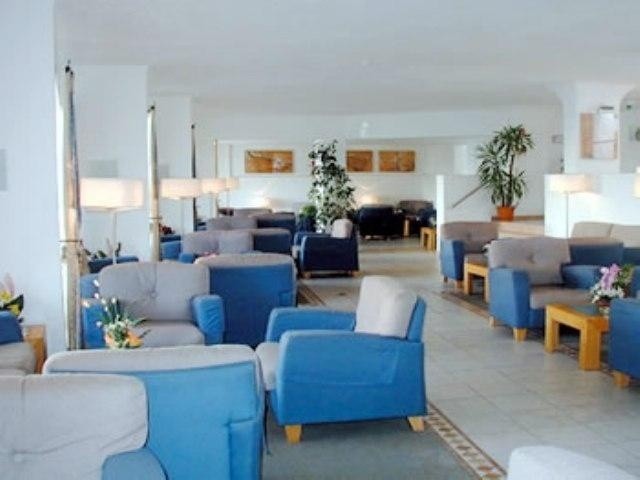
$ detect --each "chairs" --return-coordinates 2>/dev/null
[292,199,440,277]
[0,197,298,374]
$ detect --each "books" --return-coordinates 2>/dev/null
[190,250,219,264]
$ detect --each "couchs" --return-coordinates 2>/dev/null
[257,272,429,441]
[1,375,165,480]
[43,342,263,480]
[440,217,640,390]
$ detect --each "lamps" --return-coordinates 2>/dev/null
[202,172,226,215]
[545,170,592,237]
[218,175,240,219]
[159,176,198,237]
[79,176,144,266]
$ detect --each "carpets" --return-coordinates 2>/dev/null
[257,277,508,479]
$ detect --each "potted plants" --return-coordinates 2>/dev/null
[477,116,535,218]
